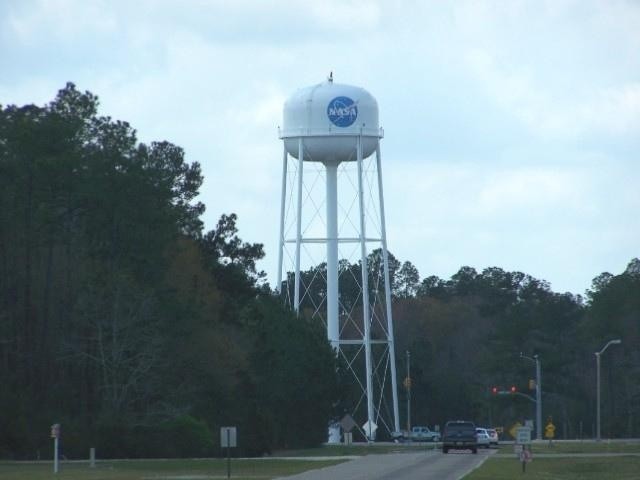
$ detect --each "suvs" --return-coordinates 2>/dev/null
[442,420,477,454]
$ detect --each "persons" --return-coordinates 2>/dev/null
[545,419,556,449]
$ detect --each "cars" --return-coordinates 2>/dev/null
[476,427,498,449]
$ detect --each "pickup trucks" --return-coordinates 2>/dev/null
[400,426,442,442]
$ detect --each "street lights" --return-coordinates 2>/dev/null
[594,339,622,440]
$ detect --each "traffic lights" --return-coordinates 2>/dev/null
[510,386,518,392]
[491,387,497,394]
